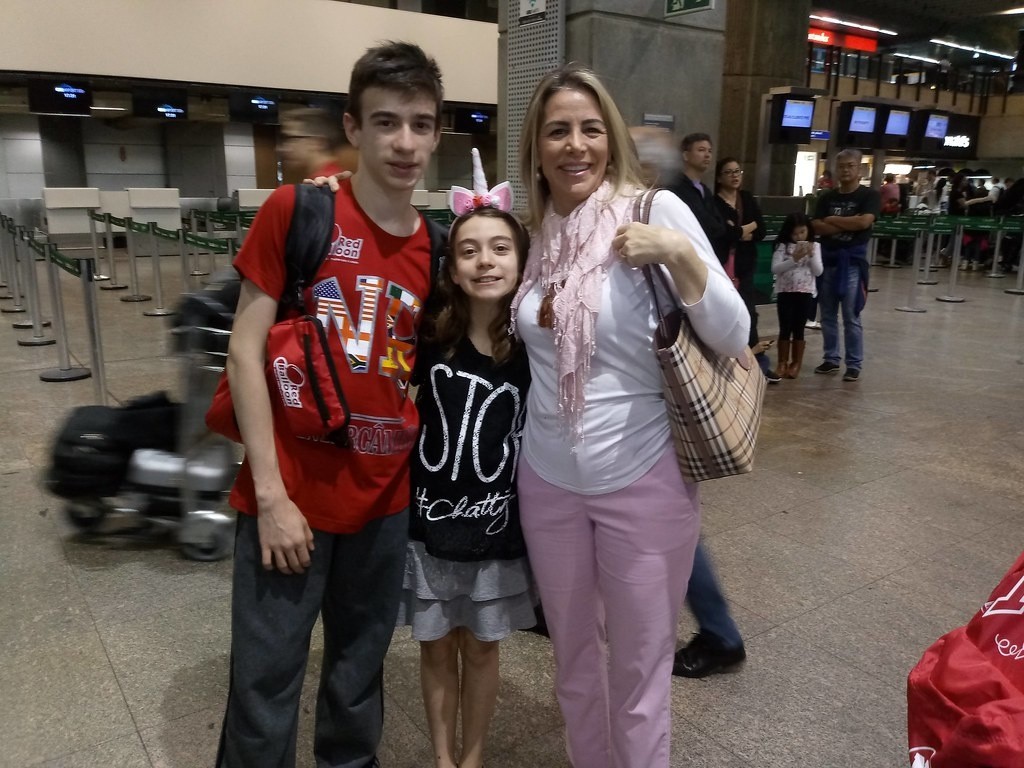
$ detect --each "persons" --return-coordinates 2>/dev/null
[817,170,834,190]
[921,178,947,214]
[622,124,746,681]
[404,207,536,768]
[212,42,443,768]
[974,179,987,199]
[881,174,899,216]
[926,171,947,211]
[771,215,824,381]
[817,151,881,382]
[941,175,975,269]
[987,177,1007,209]
[711,159,782,384]
[663,133,725,268]
[304,63,750,768]
[999,178,1014,199]
[273,107,347,187]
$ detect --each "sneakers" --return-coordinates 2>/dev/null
[815,362,840,373]
[844,369,860,381]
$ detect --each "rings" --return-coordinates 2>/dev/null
[619,248,626,259]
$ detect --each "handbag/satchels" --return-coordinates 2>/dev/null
[266,315,351,438]
[634,188,768,485]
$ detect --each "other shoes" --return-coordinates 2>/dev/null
[520,610,549,637]
[765,369,782,381]
[671,633,746,678]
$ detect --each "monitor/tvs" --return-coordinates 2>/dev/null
[925,114,949,138]
[885,110,910,135]
[131,84,188,119]
[27,80,90,117]
[781,99,814,128]
[227,91,279,126]
[453,108,491,135]
[849,107,876,133]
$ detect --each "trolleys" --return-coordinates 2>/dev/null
[65,324,240,561]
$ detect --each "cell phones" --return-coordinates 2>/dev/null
[765,340,774,346]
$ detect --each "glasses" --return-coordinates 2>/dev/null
[839,163,858,170]
[721,168,744,177]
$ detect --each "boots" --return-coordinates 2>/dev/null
[958,260,968,270]
[776,340,790,378]
[972,261,978,271]
[788,341,806,379]
